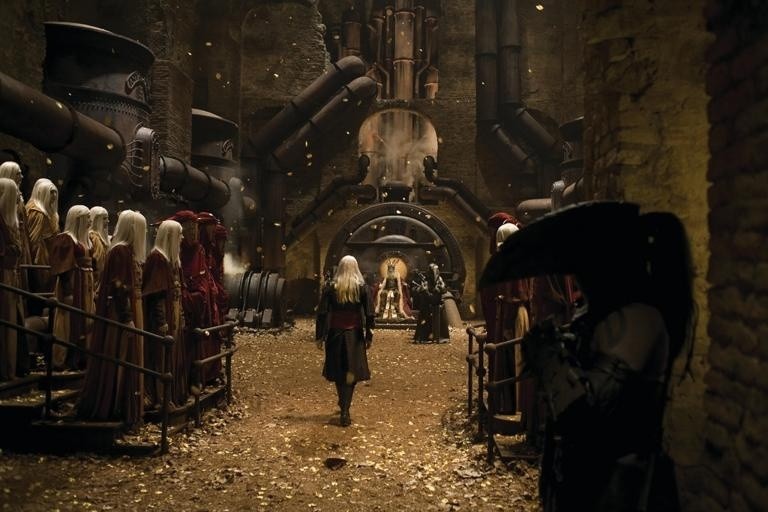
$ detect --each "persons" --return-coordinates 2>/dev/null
[370,260,415,323]
[428,264,451,345]
[0,160,235,438]
[316,253,371,425]
[477,210,531,418]
[410,263,441,344]
